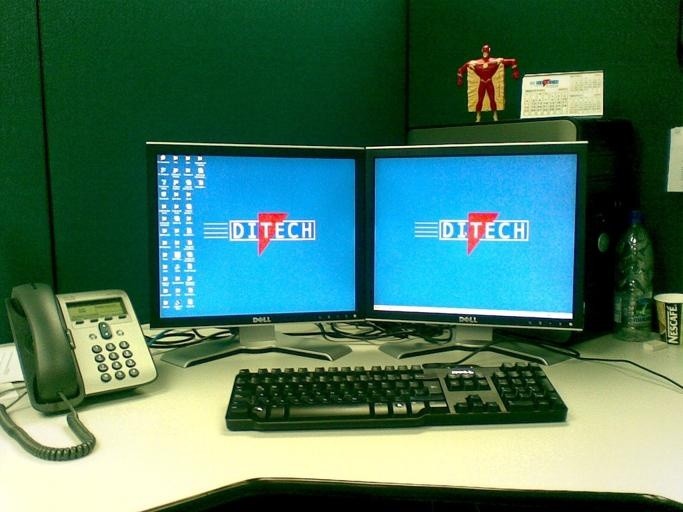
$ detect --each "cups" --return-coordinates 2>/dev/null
[653,292,681,344]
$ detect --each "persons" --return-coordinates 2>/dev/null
[458,43,519,123]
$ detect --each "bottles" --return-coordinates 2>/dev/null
[613,211,653,342]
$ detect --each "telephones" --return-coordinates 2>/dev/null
[5,282,158,415]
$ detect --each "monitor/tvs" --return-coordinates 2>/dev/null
[146,141,364,369]
[368,139,589,366]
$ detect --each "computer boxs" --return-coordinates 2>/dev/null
[408,118,632,344]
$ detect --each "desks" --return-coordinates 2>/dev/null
[0,322,683,512]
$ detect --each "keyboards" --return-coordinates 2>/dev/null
[226,362,568,429]
